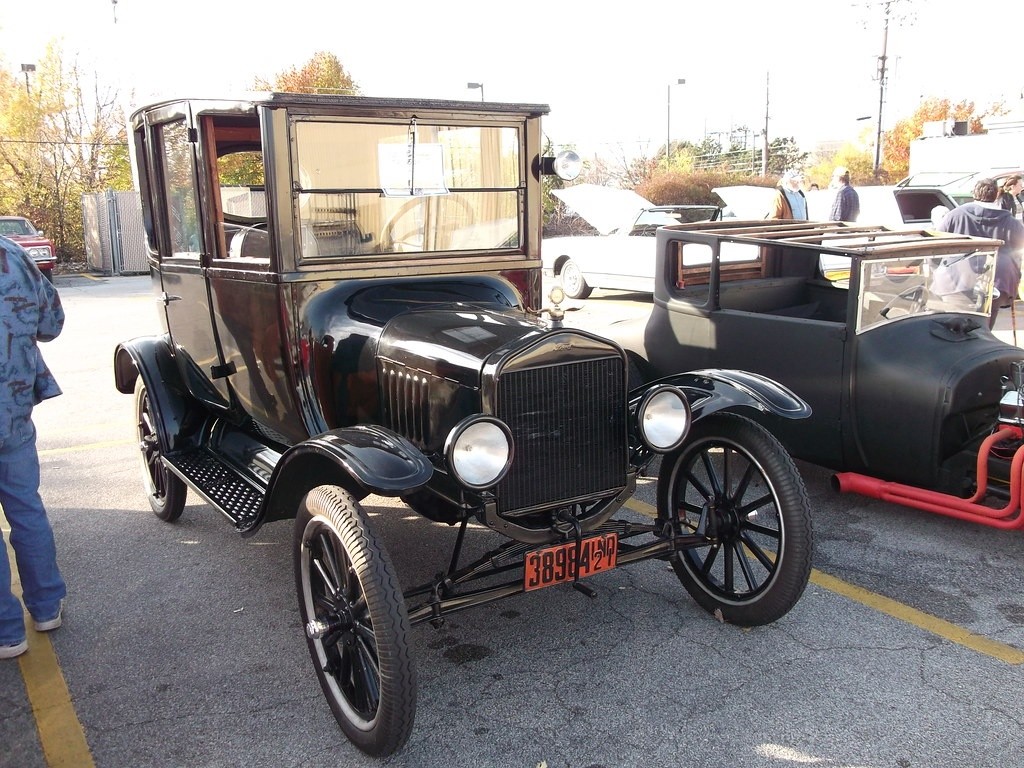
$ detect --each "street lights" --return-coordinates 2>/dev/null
[668,76,686,171]
[20,62,34,98]
[468,83,485,100]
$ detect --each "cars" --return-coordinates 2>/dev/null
[1,217,58,283]
[540,169,1024,299]
[112,92,817,760]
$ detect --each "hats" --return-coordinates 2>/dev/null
[931,205,950,226]
[832,167,848,180]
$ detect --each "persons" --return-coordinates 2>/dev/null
[0,233,68,659]
[922,172,1024,335]
[769,168,809,222]
[828,166,860,225]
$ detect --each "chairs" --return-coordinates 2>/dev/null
[228,221,322,260]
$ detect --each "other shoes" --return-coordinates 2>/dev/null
[34,599,62,631]
[0,638,28,659]
